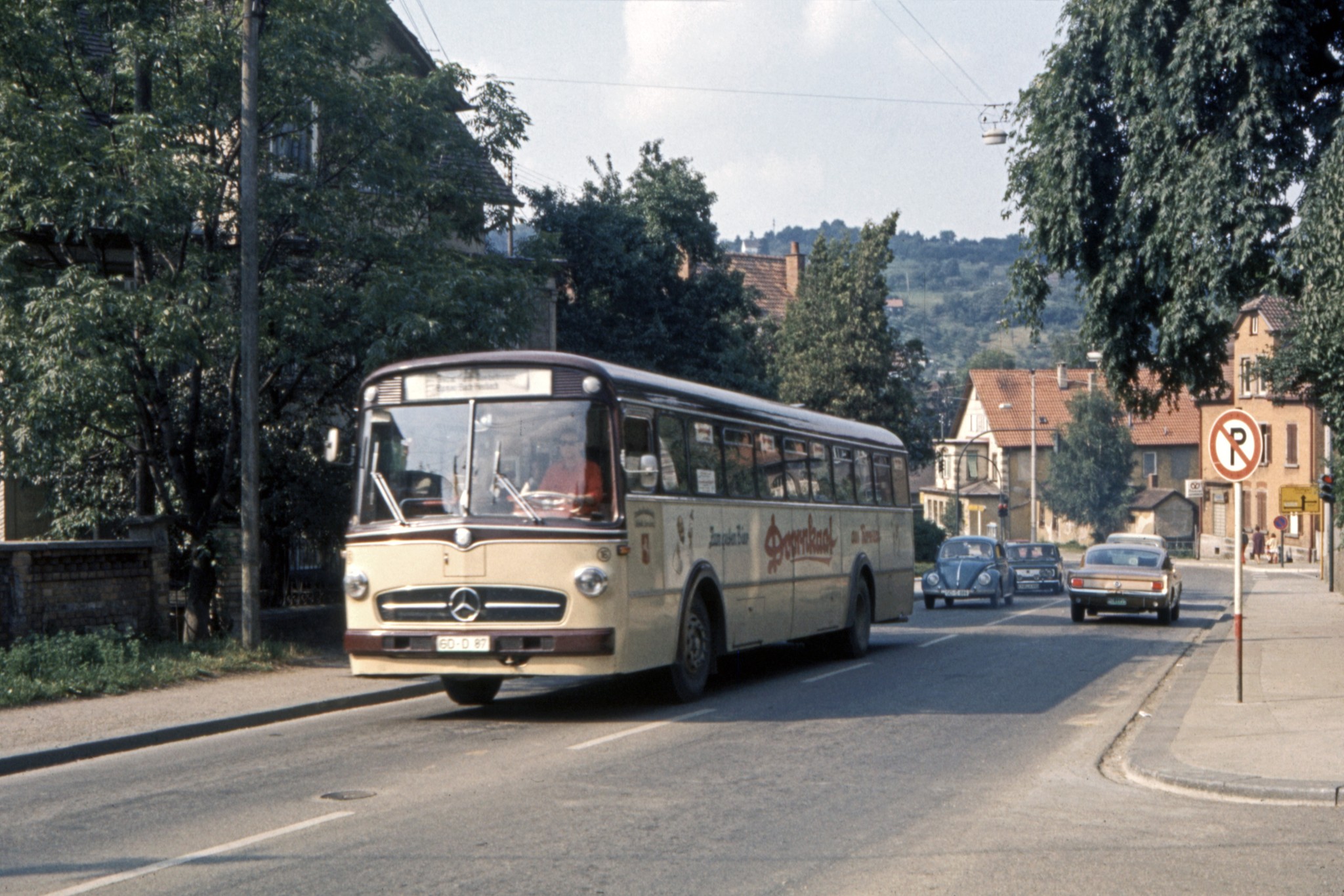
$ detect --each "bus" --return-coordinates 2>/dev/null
[325,350,915,705]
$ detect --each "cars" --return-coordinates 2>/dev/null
[1003,539,1066,596]
[1066,533,1183,624]
[922,537,1018,609]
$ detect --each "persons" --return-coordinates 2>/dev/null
[513,426,605,518]
[980,543,990,556]
[1265,533,1280,563]
[1252,525,1265,565]
[1242,528,1249,564]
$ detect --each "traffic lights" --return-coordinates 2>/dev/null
[1317,473,1335,504]
[938,458,944,472]
[998,498,1009,518]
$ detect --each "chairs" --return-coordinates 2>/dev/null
[1096,554,1113,564]
[1038,548,1054,559]
[1137,556,1157,566]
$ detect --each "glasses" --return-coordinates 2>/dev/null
[559,438,581,446]
